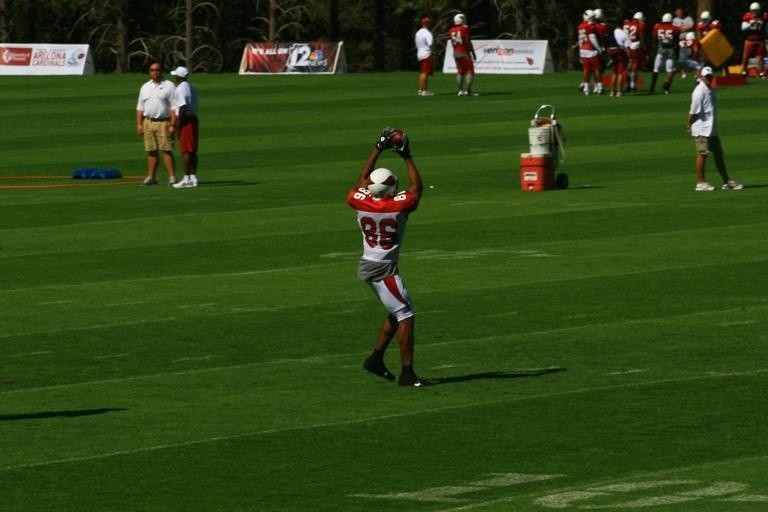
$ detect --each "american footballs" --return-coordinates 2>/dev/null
[380,127,403,149]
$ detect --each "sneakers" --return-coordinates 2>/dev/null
[140,175,198,188]
[649,84,670,94]
[399,372,435,386]
[578,83,638,97]
[721,180,744,190]
[458,90,478,96]
[418,89,435,97]
[363,357,396,381]
[695,183,715,191]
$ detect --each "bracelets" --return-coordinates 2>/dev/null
[169,124,177,128]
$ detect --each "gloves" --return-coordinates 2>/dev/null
[375,126,411,159]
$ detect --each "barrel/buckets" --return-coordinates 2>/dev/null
[529,126,550,155]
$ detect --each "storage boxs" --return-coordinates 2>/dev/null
[520,152,555,192]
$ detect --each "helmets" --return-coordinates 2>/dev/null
[454,13,466,25]
[633,11,643,19]
[700,11,711,20]
[583,8,602,21]
[367,168,397,198]
[749,2,761,15]
[662,13,672,22]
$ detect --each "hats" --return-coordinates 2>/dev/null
[170,66,189,79]
[422,17,431,25]
[700,66,713,76]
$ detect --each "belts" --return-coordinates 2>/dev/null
[146,118,170,122]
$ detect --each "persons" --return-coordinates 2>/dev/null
[572,0,767,102]
[687,67,748,194]
[134,62,181,187]
[414,16,440,99]
[446,14,480,99]
[344,123,437,388]
[164,66,203,189]
